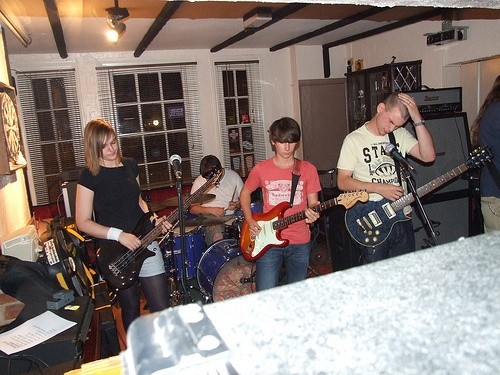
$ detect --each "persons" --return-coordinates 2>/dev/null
[473,76,500,234]
[336,91,436,266]
[188,155,245,246]
[75,118,172,335]
[240,116,322,292]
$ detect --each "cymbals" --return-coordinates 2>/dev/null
[161,193,216,207]
[145,202,168,212]
[185,215,234,226]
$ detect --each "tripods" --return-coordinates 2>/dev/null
[171,177,209,304]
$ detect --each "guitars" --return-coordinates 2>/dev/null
[344,148,493,248]
[97,168,224,289]
[240,189,369,262]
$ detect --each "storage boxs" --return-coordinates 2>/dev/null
[120,232,500,375]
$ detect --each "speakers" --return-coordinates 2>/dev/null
[426,31,463,46]
[403,112,475,252]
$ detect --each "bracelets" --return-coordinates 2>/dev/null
[152,217,160,226]
[413,118,425,127]
[107,227,124,242]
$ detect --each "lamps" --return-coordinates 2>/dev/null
[105,0,130,44]
[243,7,273,28]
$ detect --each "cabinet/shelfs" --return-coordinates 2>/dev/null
[0,81,27,174]
[298,59,422,191]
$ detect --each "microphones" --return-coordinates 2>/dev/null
[385,144,418,174]
[240,277,256,284]
[168,154,182,177]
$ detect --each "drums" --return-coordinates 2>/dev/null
[197,239,257,304]
[162,227,206,281]
